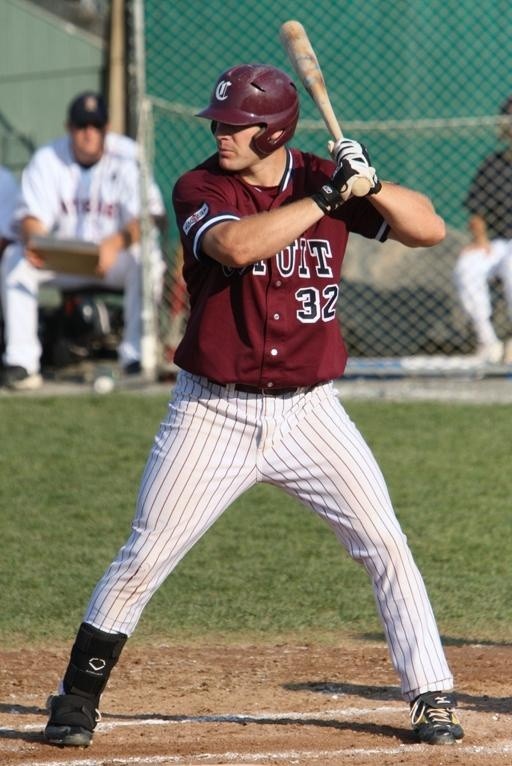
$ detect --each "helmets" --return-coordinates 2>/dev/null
[195,64,300,156]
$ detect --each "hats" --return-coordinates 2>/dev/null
[71,93,107,127]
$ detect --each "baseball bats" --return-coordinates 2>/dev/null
[279,20,371,198]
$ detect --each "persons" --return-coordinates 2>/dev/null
[450,94,511,374]
[40,57,467,754]
[1,89,167,395]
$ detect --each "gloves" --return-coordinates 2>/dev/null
[317,134,382,206]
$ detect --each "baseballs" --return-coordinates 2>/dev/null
[90,375,115,394]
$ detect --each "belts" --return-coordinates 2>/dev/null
[208,374,327,396]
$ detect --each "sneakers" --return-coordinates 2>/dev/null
[123,359,142,377]
[4,366,40,388]
[45,692,101,746]
[409,692,464,746]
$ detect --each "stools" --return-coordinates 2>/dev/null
[61,283,123,360]
